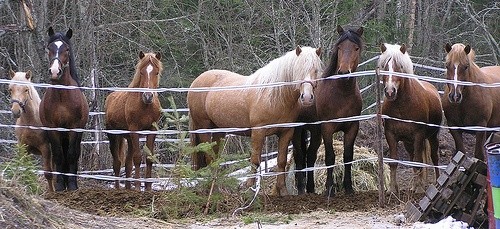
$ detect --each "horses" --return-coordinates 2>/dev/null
[7,68,55,191]
[186,44,322,197]
[376,42,443,200]
[106,50,163,192]
[443,42,500,160]
[39,26,89,190]
[292,25,364,197]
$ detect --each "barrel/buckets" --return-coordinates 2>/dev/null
[483,142,500,229]
